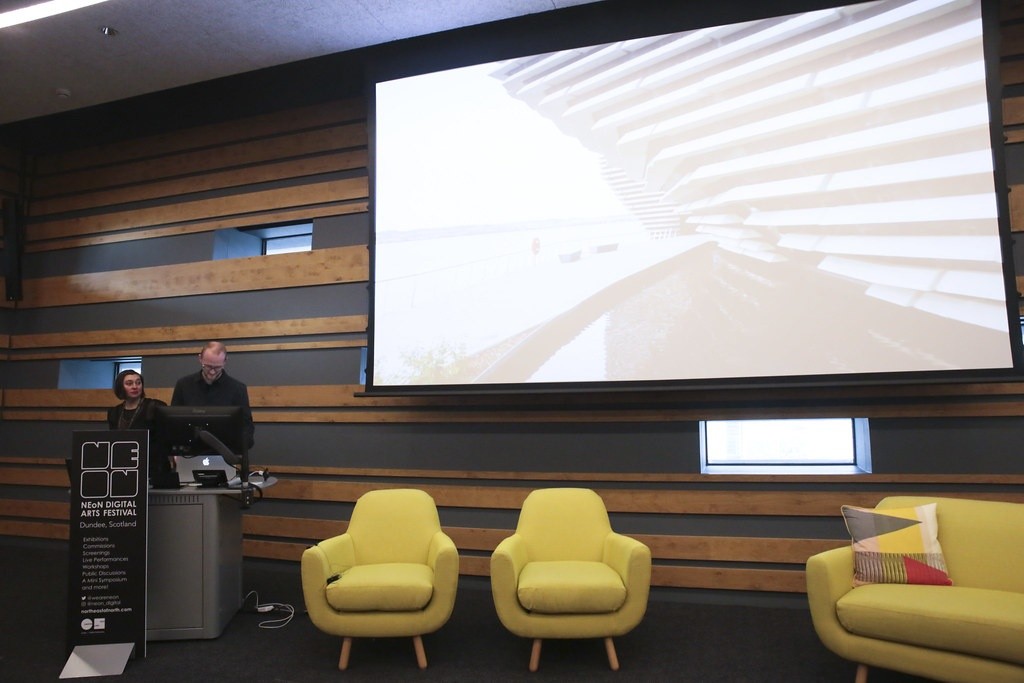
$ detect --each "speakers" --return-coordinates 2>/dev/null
[1,196,24,301]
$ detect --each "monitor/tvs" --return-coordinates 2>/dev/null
[154,405,244,455]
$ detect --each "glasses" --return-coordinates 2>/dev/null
[200,360,224,371]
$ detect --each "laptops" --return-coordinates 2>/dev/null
[171,456,237,487]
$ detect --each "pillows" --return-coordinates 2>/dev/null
[839,500,955,591]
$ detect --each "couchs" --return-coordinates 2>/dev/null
[801,490,1024,682]
[299,484,462,675]
[489,486,656,670]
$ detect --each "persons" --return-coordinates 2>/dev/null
[170,341,255,456]
[107,369,172,479]
